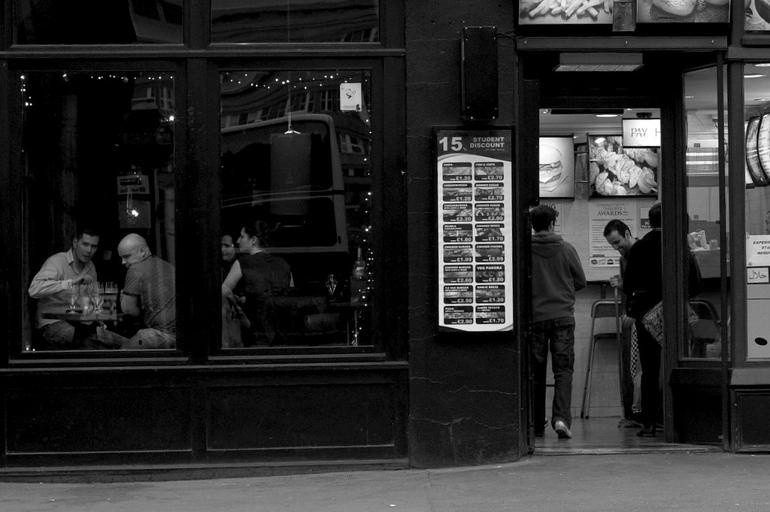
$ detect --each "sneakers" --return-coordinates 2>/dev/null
[555,419,572,439]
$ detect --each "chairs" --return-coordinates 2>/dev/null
[685,297,721,344]
[580,300,622,419]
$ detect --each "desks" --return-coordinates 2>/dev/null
[41,310,131,350]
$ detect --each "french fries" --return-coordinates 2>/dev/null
[529,0,609,17]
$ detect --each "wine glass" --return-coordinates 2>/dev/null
[65,280,119,315]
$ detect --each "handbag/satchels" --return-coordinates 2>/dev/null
[641,299,700,350]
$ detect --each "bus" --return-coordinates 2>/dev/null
[216,115,366,302]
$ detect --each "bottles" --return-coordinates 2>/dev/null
[349,246,370,305]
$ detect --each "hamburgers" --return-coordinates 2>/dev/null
[539,146,562,183]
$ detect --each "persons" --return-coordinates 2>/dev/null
[28,225,105,361]
[600,217,643,429]
[526,201,587,440]
[220,218,297,346]
[114,231,175,350]
[621,201,705,438]
[215,230,239,265]
